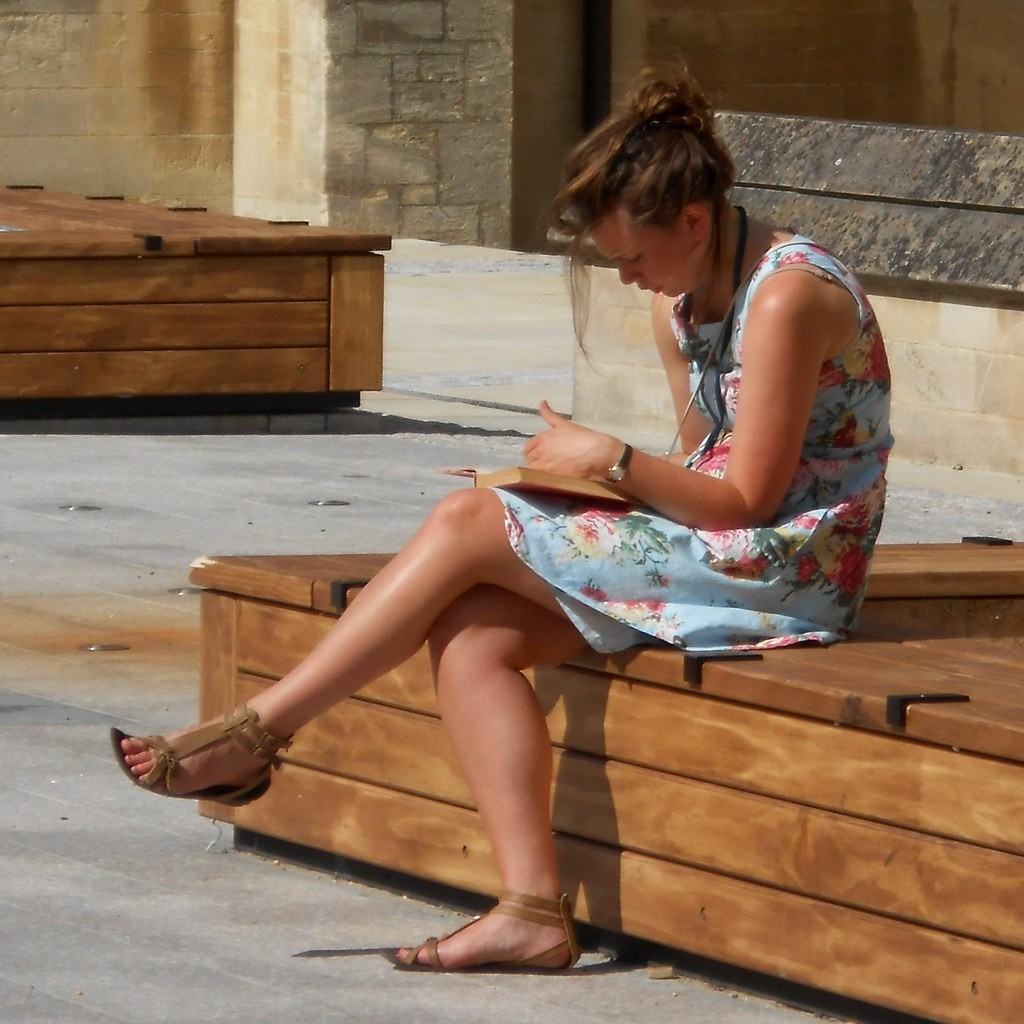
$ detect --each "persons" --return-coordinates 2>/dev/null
[107,62,896,975]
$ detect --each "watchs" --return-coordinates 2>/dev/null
[604,443,634,485]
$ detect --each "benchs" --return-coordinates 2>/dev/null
[188,542,1024,1024]
[0,184,391,420]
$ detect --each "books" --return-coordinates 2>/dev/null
[437,466,630,506]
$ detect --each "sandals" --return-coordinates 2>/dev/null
[109,700,295,807]
[378,890,580,974]
